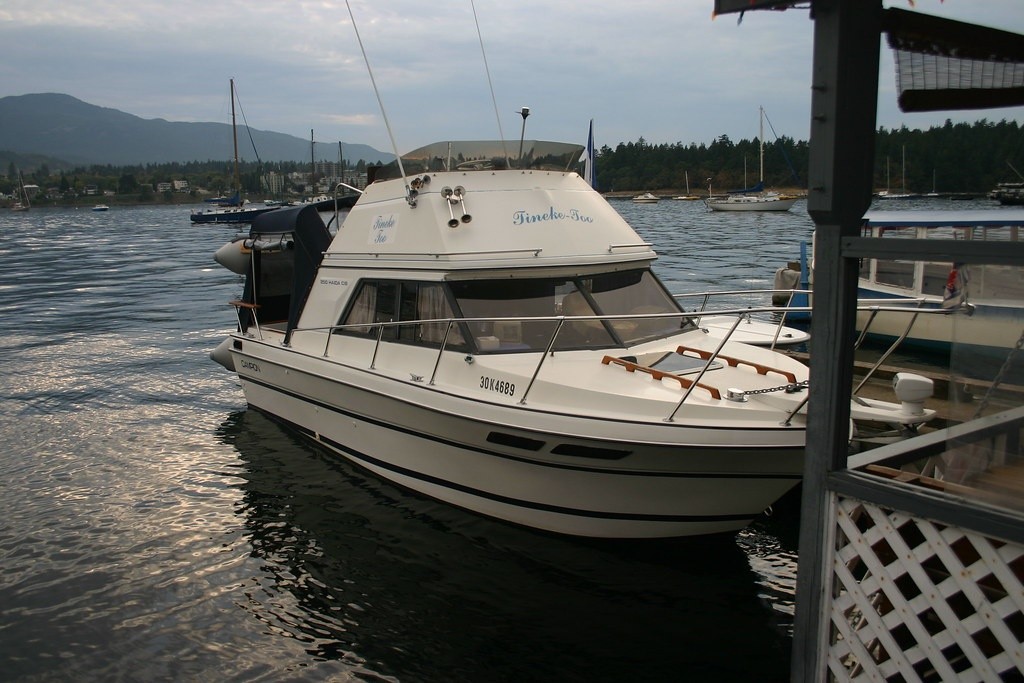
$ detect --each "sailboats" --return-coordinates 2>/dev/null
[704,102,809,212]
[189,76,349,224]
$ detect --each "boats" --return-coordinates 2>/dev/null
[849,395,940,425]
[672,192,702,201]
[632,190,662,204]
[208,0,858,543]
[772,209,1024,372]
[686,310,812,347]
